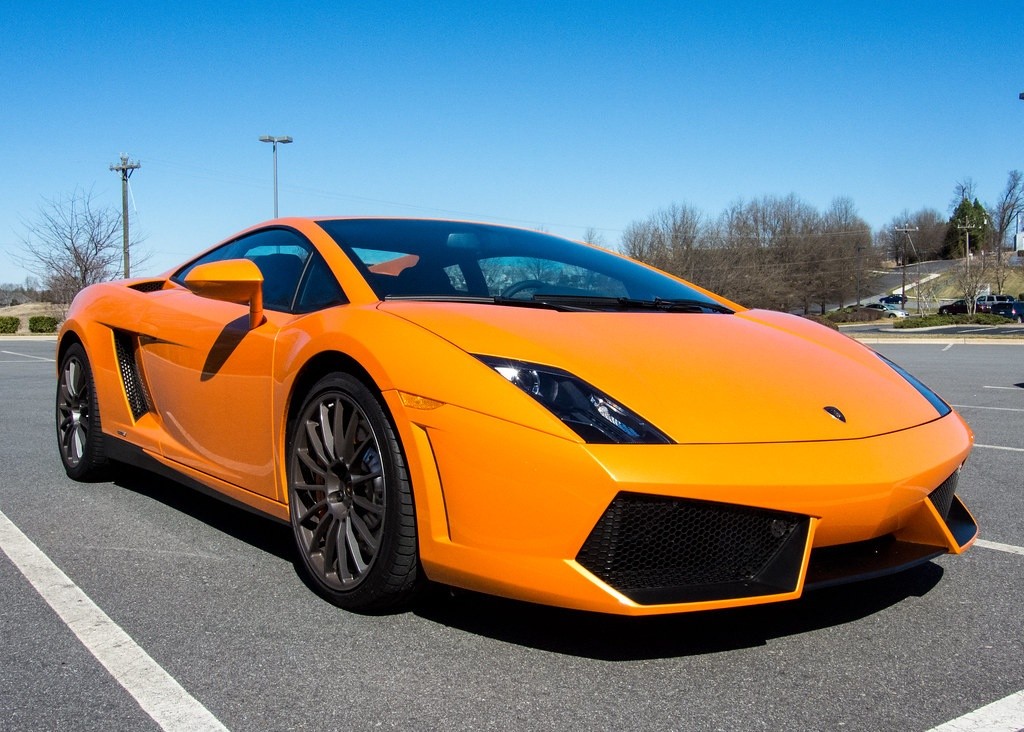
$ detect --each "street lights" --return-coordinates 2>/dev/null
[260,135,293,222]
[110,152,142,278]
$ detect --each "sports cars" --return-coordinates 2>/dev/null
[53,212,982,617]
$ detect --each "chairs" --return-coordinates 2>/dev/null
[254,254,302,305]
[397,267,458,297]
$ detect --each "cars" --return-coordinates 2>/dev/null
[975,294,1014,305]
[938,299,982,315]
[866,303,910,320]
[879,293,907,304]
[990,301,1024,323]
